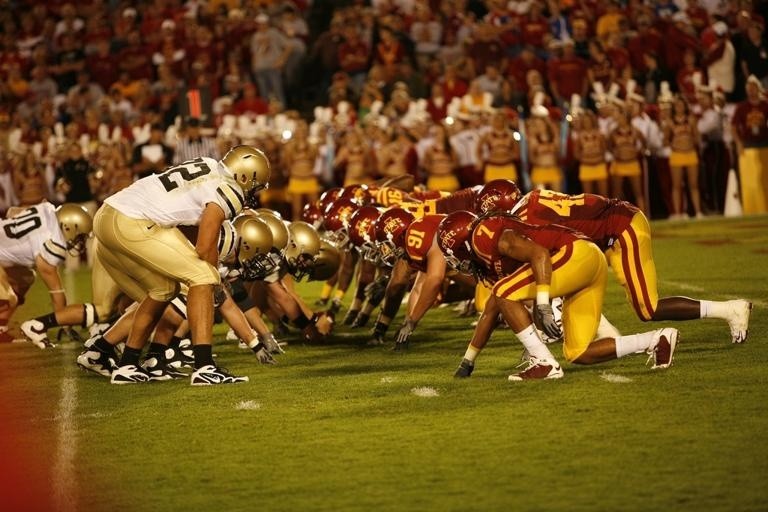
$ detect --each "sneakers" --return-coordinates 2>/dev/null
[503,355,565,382]
[238,328,259,349]
[649,326,681,371]
[694,211,706,220]
[163,338,219,369]
[18,315,58,351]
[140,349,191,381]
[723,294,754,346]
[668,210,690,224]
[226,327,239,341]
[83,302,130,355]
[190,364,250,386]
[108,361,170,385]
[542,296,564,344]
[74,344,121,379]
[704,198,735,216]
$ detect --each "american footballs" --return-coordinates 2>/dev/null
[303,311,336,343]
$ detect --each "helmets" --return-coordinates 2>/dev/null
[220,144,272,211]
[55,201,94,258]
[476,177,524,216]
[342,184,372,208]
[256,206,290,262]
[320,187,346,219]
[285,220,321,284]
[302,200,320,226]
[230,214,278,283]
[373,207,417,267]
[304,234,342,282]
[436,208,486,285]
[323,200,358,253]
[347,206,383,265]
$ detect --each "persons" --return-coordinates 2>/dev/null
[1,1,767,386]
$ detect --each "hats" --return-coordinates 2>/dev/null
[160,18,176,30]
[121,8,137,19]
[150,122,166,132]
[709,17,729,36]
[254,11,271,23]
[187,117,201,129]
